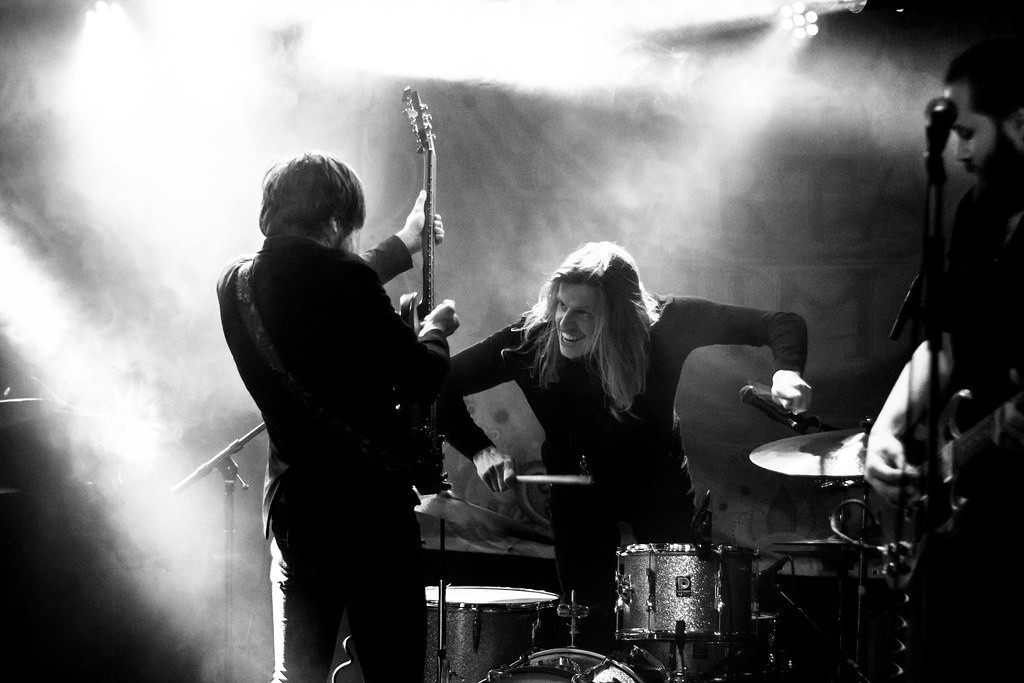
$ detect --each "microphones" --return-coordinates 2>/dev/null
[740,385,810,434]
[757,557,791,585]
[924,97,959,179]
[699,493,712,542]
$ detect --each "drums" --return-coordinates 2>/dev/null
[476,647,643,683]
[633,611,778,678]
[424,584,561,683]
[614,542,754,643]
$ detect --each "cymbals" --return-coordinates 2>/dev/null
[748,428,869,477]
[771,549,858,557]
[771,537,851,547]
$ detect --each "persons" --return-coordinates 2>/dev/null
[437,241,813,658]
[865,40,1024,683]
[215,153,460,683]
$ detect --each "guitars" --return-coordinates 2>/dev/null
[870,388,1024,589]
[399,84,455,498]
